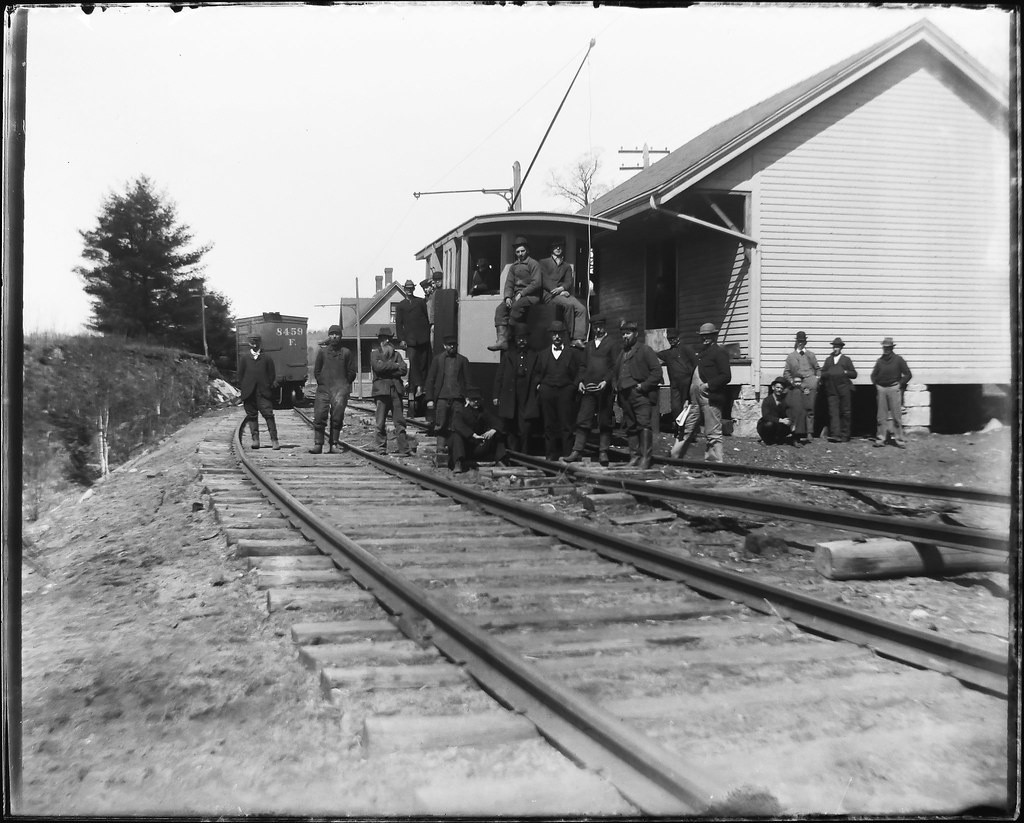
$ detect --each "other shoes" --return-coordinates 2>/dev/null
[873,442,885,447]
[767,433,850,448]
[251,439,416,457]
[454,461,462,472]
[569,339,586,349]
[498,461,507,468]
[895,443,906,449]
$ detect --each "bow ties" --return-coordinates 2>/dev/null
[554,347,562,350]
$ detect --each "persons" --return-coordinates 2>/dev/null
[371,327,417,456]
[236,335,281,451]
[538,320,581,476]
[447,392,505,472]
[756,376,795,445]
[783,331,821,443]
[426,338,473,454]
[561,313,619,464]
[307,325,357,453]
[655,323,731,464]
[870,337,912,449]
[468,256,500,296]
[420,272,444,352]
[820,338,858,442]
[487,236,542,350]
[535,239,588,348]
[612,320,663,471]
[395,280,430,400]
[489,322,543,466]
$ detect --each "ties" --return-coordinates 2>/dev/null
[800,351,804,356]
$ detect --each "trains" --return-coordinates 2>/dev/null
[233,311,309,410]
[410,160,621,401]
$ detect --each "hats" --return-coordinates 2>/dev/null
[795,332,807,340]
[830,338,845,346]
[515,313,719,338]
[247,332,260,340]
[772,377,786,388]
[881,338,896,347]
[377,327,393,337]
[328,325,342,336]
[401,272,443,288]
[512,237,528,248]
[468,387,482,399]
[444,335,457,344]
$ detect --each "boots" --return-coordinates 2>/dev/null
[488,326,509,350]
[563,428,652,469]
